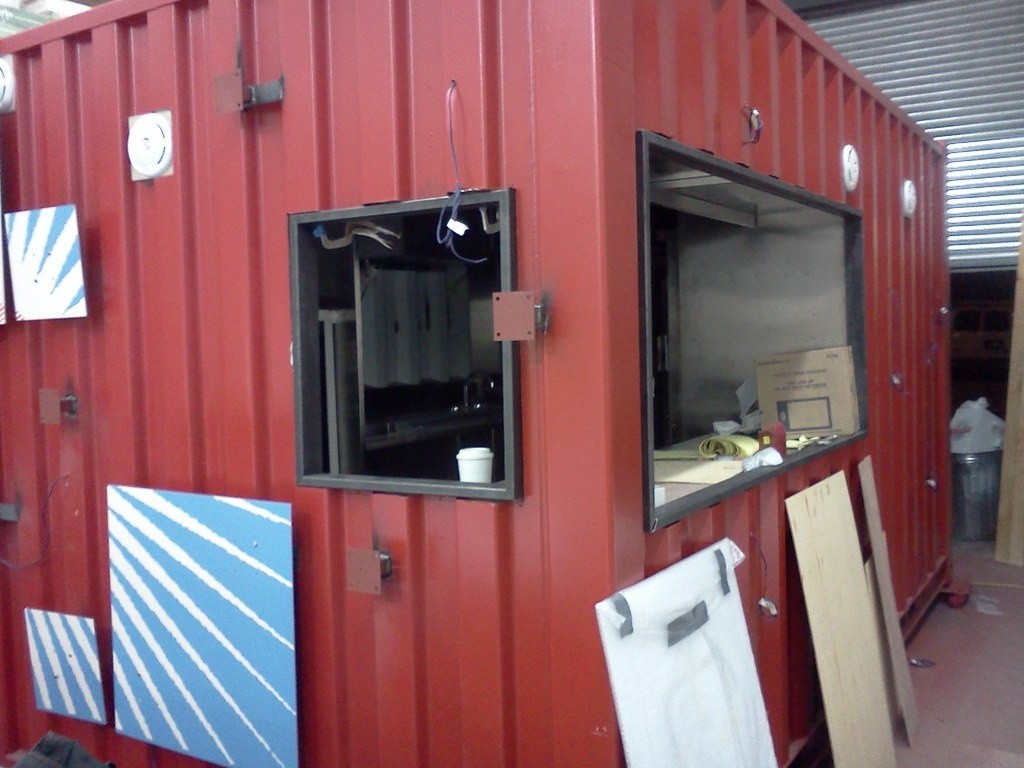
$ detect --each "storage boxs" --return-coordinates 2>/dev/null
[755,346,860,439]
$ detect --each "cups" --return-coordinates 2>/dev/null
[456,447,494,482]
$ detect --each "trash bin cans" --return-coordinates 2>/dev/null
[949,449,1002,542]
